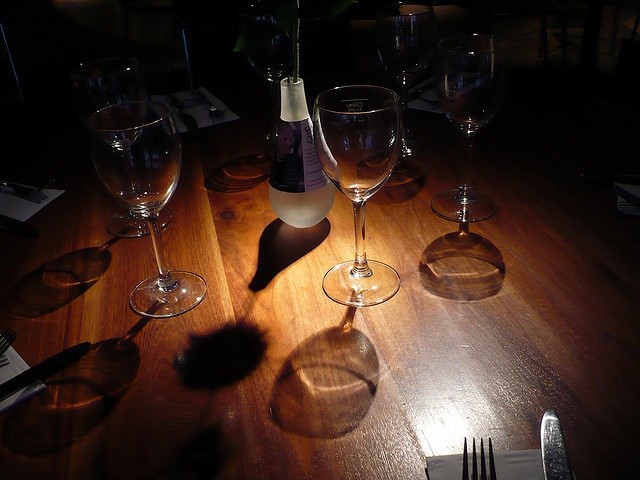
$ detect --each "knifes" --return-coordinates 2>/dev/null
[1,342,93,401]
[540,408,574,480]
[167,95,197,131]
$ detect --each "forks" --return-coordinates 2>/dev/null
[462,437,497,480]
[195,89,226,124]
[0,180,49,204]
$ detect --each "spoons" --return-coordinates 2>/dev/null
[1,325,17,360]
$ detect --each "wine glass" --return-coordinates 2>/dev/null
[430,33,501,223]
[312,86,401,308]
[89,59,173,238]
[247,9,294,144]
[375,4,435,158]
[89,100,208,318]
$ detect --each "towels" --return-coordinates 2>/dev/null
[0,180,65,222]
[615,175,640,216]
[149,87,239,136]
[408,89,445,114]
[0,334,46,414]
[427,450,577,480]
